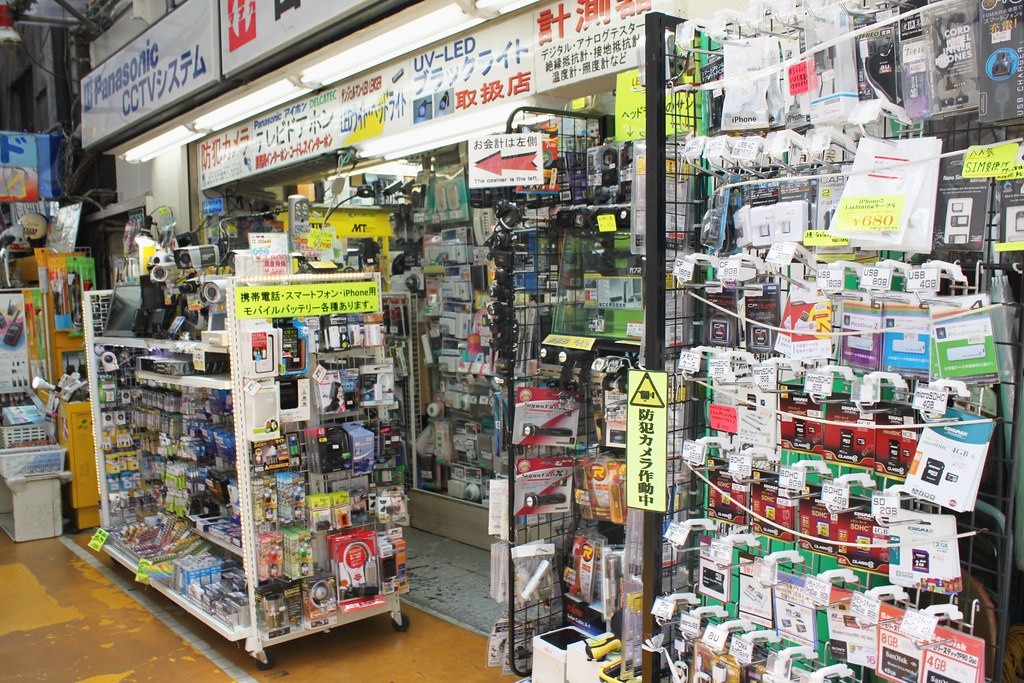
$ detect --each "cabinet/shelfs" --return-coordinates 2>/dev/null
[77,271,414,672]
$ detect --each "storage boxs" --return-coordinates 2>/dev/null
[0,0,1024,683]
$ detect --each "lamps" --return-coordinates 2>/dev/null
[297,0,489,87]
[468,0,540,17]
[122,122,210,164]
[195,73,316,133]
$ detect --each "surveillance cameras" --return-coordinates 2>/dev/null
[399,180,416,195]
[385,181,403,195]
